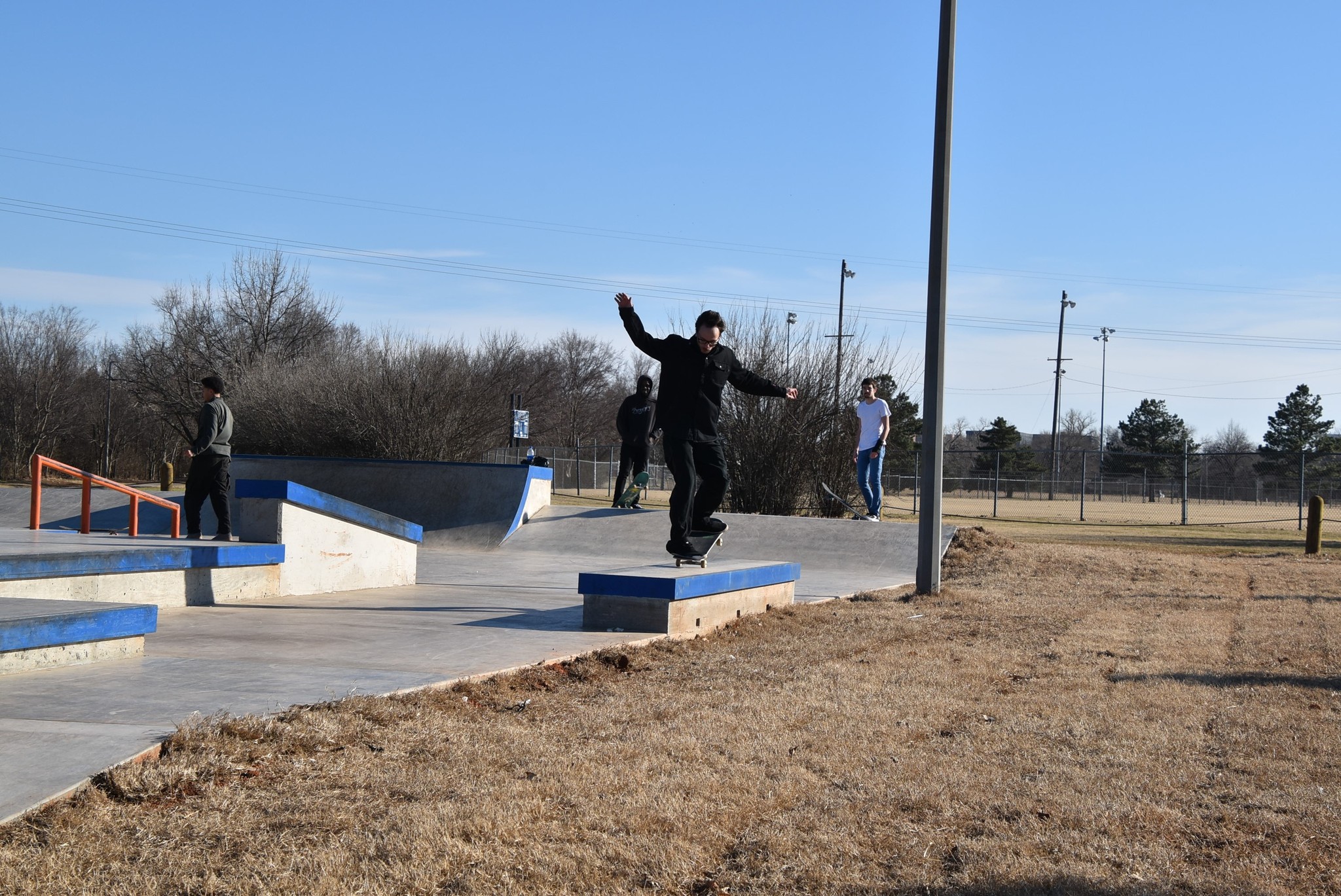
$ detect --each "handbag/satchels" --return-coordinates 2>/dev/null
[534,456,550,467]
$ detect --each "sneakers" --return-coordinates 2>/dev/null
[672,542,706,560]
[691,521,729,532]
[863,513,880,522]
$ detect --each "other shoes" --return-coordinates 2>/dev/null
[211,532,232,541]
[185,532,203,539]
[631,503,644,509]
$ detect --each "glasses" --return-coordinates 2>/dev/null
[696,329,721,346]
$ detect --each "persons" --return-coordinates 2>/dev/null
[611,375,662,508]
[184,376,234,540]
[615,292,798,554]
[853,378,892,522]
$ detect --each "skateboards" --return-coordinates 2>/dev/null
[58,526,130,536]
[821,482,871,522]
[612,471,649,508]
[672,517,723,568]
